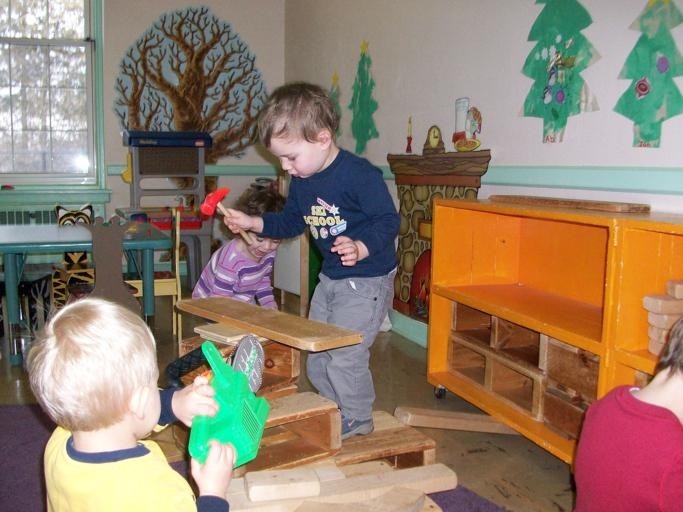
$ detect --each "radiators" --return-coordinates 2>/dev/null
[0,192,111,265]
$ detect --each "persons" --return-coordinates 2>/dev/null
[191,187,281,327]
[570,312,682,511]
[214,80,400,441]
[24,297,237,512]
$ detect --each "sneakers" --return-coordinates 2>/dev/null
[341,414,374,440]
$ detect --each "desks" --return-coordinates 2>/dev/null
[115,206,214,293]
[0,222,174,368]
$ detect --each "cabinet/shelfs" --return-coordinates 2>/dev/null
[424,194,683,476]
